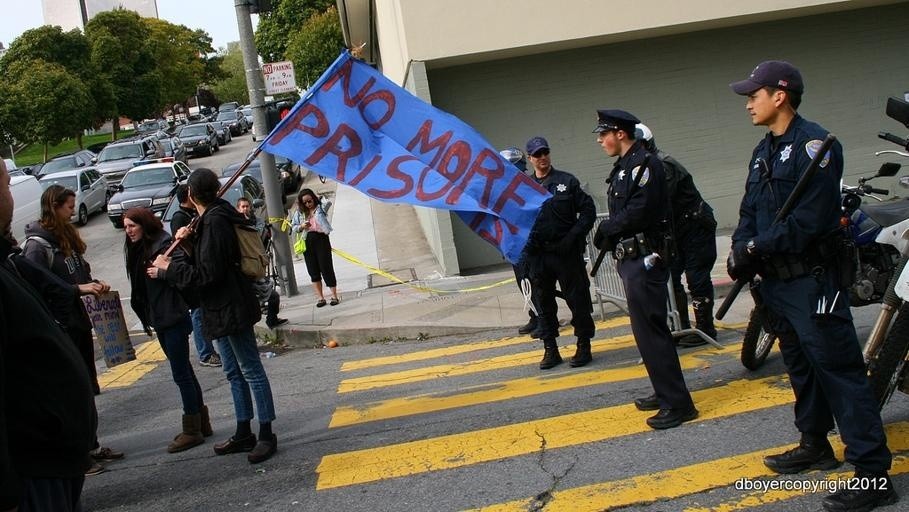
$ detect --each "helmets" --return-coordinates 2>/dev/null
[499,148,527,172]
[634,124,656,152]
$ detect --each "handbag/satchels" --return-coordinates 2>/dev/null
[252,280,273,301]
[294,236,306,255]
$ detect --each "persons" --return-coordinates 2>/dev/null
[0,156,289,512]
[726,59,898,512]
[594,110,699,429]
[515,137,596,369]
[497,148,561,337]
[291,188,338,308]
[634,122,719,346]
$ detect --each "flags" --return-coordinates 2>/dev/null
[260,51,553,265]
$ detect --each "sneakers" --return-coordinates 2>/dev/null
[199,353,222,366]
[269,319,288,329]
[764,432,838,474]
[634,393,663,410]
[647,408,699,428]
[822,471,896,512]
[317,298,338,307]
[92,447,124,462]
[83,461,105,476]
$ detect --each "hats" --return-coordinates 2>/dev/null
[592,109,641,132]
[729,61,803,95]
[526,137,549,154]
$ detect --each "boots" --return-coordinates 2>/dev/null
[174,405,212,441]
[540,338,561,369]
[570,337,592,366]
[166,413,204,452]
[518,307,543,338]
[670,288,716,346]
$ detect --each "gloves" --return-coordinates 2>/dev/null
[594,220,622,251]
[727,240,747,280]
[561,227,584,247]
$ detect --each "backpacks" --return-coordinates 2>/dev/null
[231,223,269,278]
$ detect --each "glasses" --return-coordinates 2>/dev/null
[532,148,549,158]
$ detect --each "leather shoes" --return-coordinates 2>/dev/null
[249,434,277,463]
[214,433,256,454]
